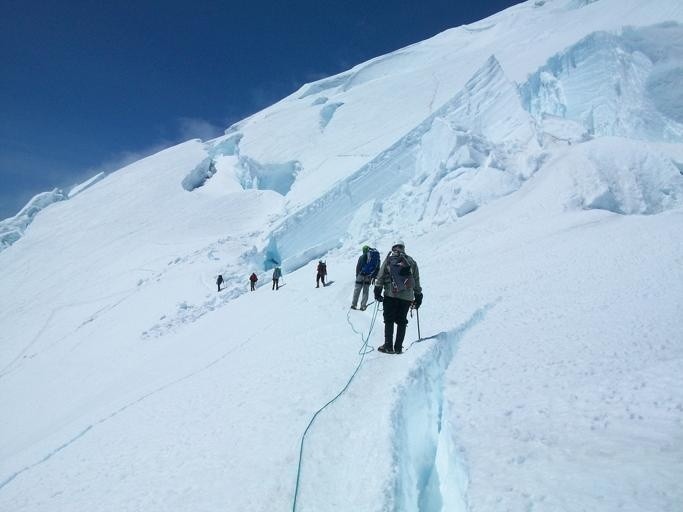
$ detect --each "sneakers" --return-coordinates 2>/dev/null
[378,343,402,354]
[351,305,366,311]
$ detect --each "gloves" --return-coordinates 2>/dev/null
[412,293,423,309]
[374,286,384,302]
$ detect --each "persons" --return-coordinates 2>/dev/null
[315,260,327,288]
[351,245,380,311]
[374,240,422,354]
[216,275,224,291]
[272,268,281,290]
[250,273,258,291]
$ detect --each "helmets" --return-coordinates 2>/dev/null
[391,239,405,250]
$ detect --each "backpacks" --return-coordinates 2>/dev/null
[360,248,380,275]
[386,252,412,295]
